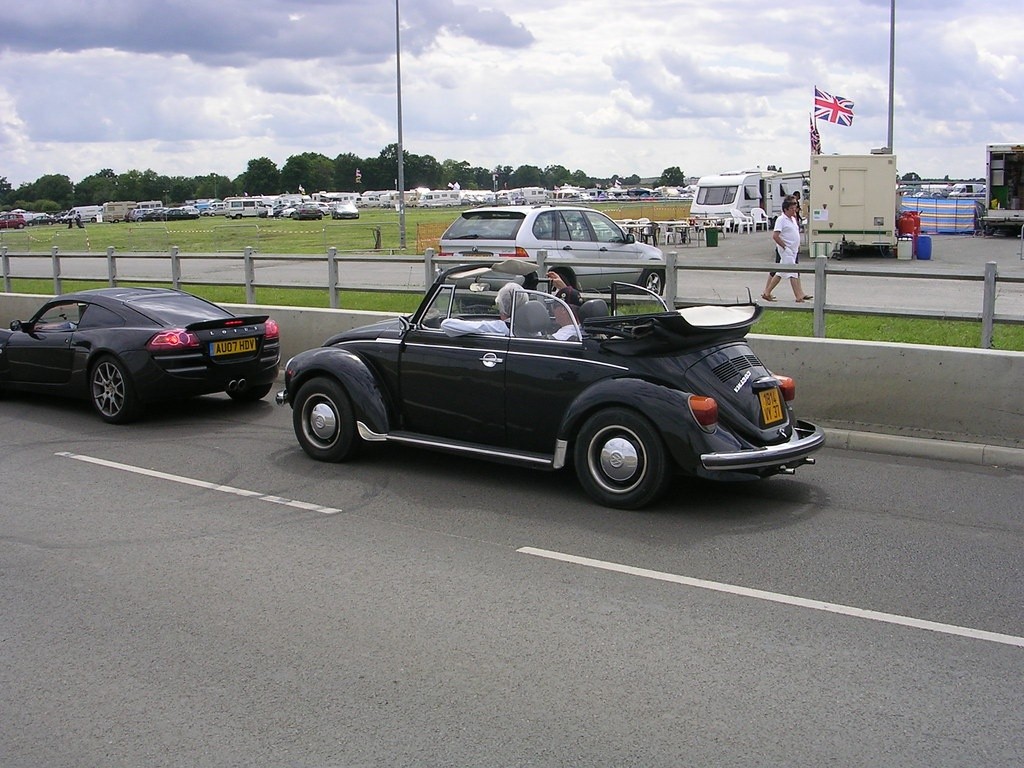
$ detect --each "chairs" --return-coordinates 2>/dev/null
[730,209,753,234]
[751,208,769,232]
[660,224,675,246]
[578,299,611,337]
[560,222,574,241]
[516,302,556,340]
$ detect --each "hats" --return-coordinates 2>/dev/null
[546,285,583,305]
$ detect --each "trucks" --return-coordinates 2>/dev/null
[982,143,1024,236]
[0,183,696,231]
[808,148,898,260]
[689,168,809,231]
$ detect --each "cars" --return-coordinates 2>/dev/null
[292,203,322,221]
[329,202,360,220]
[903,191,946,199]
[975,187,985,197]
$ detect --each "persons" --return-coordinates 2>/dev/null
[761,195,813,299]
[545,272,584,340]
[440,282,528,338]
[763,201,807,302]
[76,211,82,219]
[547,266,582,293]
[793,191,805,223]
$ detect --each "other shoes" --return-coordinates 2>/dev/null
[762,294,777,302]
[803,295,813,299]
[761,293,776,299]
[795,299,810,304]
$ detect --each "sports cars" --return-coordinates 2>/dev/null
[275,260,828,512]
[0,286,281,426]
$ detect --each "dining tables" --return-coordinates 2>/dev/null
[717,219,728,239]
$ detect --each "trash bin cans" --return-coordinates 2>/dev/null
[705,227,718,247]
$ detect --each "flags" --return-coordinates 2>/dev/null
[815,89,854,127]
[299,185,306,195]
[810,121,822,154]
[356,168,362,183]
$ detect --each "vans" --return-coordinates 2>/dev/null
[949,184,983,197]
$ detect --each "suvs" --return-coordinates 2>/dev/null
[433,202,667,297]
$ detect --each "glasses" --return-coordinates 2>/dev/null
[553,304,562,309]
[792,202,797,205]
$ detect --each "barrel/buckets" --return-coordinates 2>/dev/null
[917,235,932,259]
[897,210,920,260]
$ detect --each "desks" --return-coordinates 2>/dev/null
[617,225,661,232]
[688,217,724,227]
[672,226,691,248]
[614,220,648,239]
[670,225,703,248]
[642,222,690,245]
